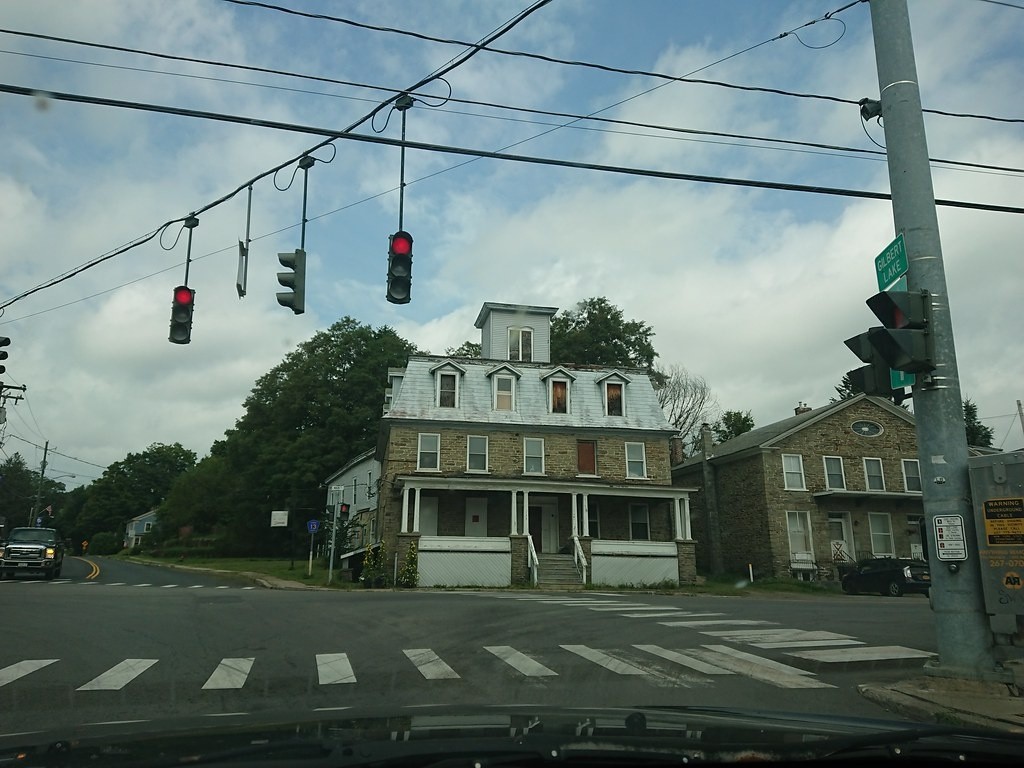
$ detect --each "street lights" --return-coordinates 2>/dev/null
[31,475,76,528]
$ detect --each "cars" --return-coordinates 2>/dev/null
[841,559,931,598]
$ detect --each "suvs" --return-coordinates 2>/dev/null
[0,527,65,580]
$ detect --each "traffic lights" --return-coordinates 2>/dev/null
[337,503,350,521]
[867,290,936,373]
[386,230,413,304]
[0,336,11,374]
[167,286,196,345]
[323,504,335,522]
[844,326,892,398]
[275,249,306,315]
